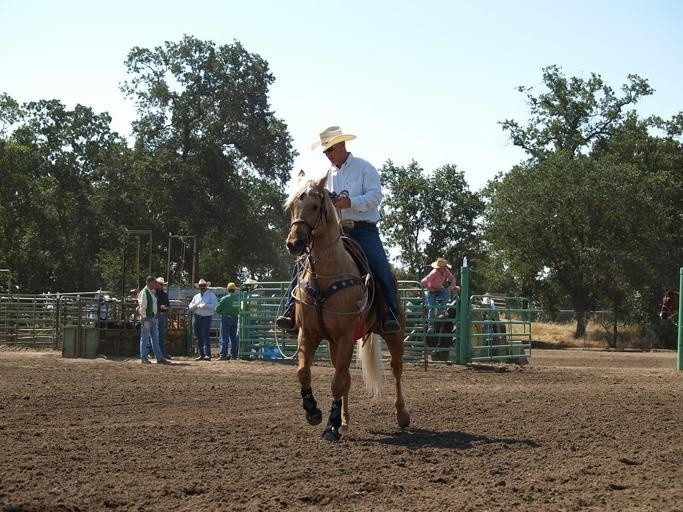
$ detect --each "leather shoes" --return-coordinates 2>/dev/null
[383,313,401,333]
[215,354,227,361]
[142,358,151,363]
[276,312,300,340]
[157,359,171,364]
[195,354,211,360]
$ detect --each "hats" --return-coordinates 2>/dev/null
[431,257,452,269]
[483,292,494,298]
[194,278,211,288]
[242,278,258,288]
[309,124,357,154]
[226,282,236,289]
[156,277,169,284]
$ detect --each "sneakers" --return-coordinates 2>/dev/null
[427,328,434,334]
[439,314,445,319]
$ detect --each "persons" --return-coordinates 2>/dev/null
[124,275,171,364]
[482,293,496,341]
[275,127,401,334]
[189,278,240,361]
[421,258,456,334]
[89,290,110,326]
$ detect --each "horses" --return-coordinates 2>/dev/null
[283,170,410,439]
